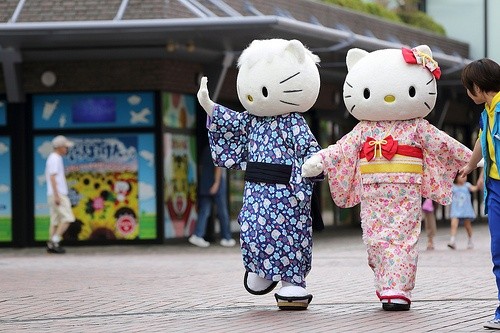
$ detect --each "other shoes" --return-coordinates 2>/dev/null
[188,234,210,248]
[483,319,500,329]
[448,242,456,249]
[427,240,434,250]
[467,239,473,248]
[48,240,67,254]
[220,238,236,247]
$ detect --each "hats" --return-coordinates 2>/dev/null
[53,135,74,149]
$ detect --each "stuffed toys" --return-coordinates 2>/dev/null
[197,38,324,310]
[301,45,484,312]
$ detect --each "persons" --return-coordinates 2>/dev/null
[458,58,500,330]
[425,209,437,250]
[188,131,236,248]
[45,136,75,254]
[446,167,484,250]
